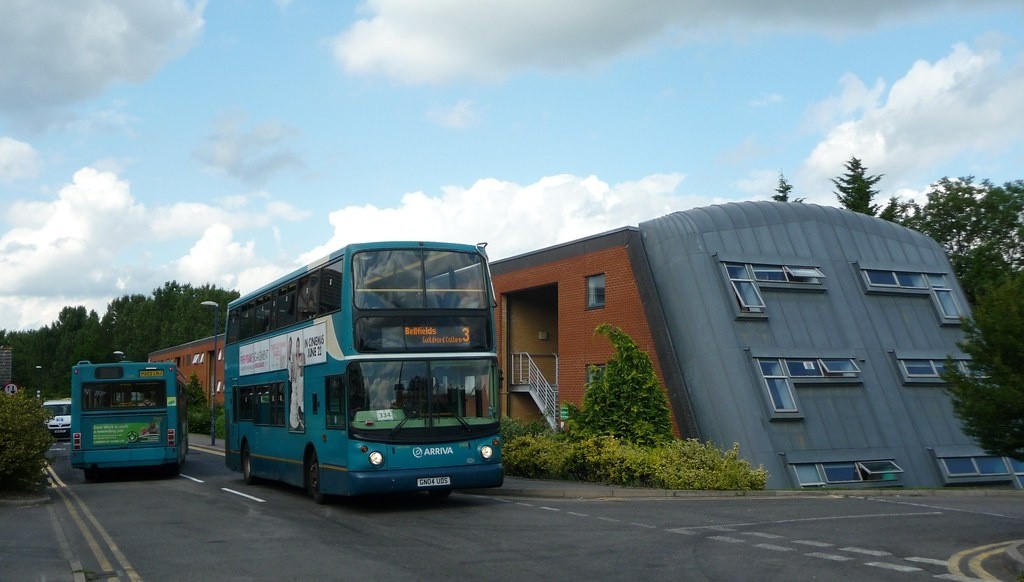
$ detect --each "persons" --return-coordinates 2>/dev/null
[97,389,157,410]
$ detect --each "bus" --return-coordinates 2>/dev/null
[71,360,188,479]
[223,239,505,506]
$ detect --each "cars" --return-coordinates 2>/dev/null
[42,398,72,439]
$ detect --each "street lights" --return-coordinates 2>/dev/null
[200,301,218,446]
[113,351,124,361]
[35,366,42,390]
